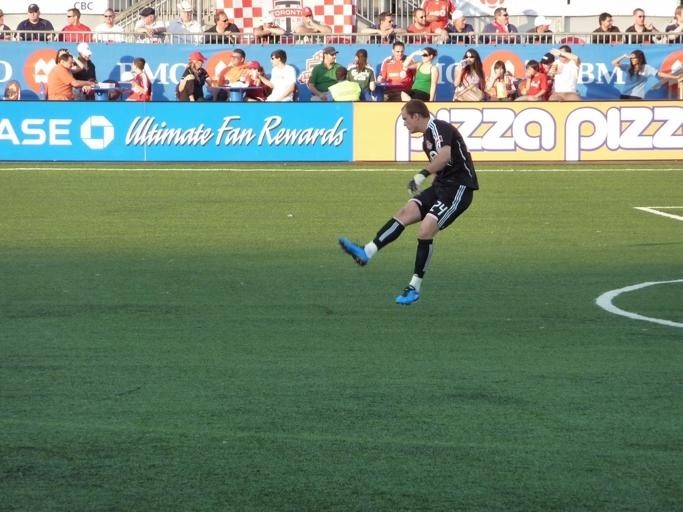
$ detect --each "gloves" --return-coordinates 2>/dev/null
[407,172,427,195]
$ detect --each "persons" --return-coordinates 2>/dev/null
[338,99,478,305]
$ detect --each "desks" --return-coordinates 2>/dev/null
[91,82,129,102]
[210,84,263,101]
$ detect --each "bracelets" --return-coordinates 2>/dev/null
[419,169,430,177]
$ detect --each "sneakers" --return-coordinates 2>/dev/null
[338,236,370,267]
[396,285,420,306]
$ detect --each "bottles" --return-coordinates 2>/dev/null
[461,59,471,66]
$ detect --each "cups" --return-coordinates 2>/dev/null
[211,74,247,89]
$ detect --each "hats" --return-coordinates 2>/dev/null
[27,3,40,14]
[76,42,93,57]
[177,0,196,12]
[189,51,209,62]
[321,46,340,55]
[534,16,553,27]
[302,7,313,17]
[541,52,555,65]
[140,7,155,17]
